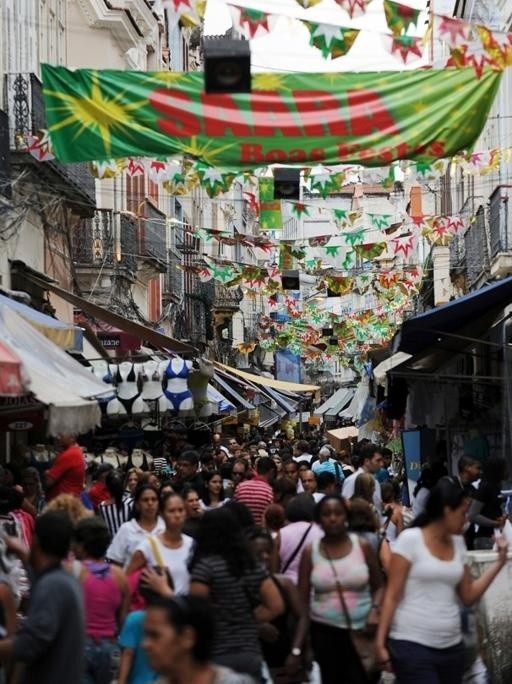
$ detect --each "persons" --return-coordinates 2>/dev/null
[1,423,512,684]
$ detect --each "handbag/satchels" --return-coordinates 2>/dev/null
[352,632,377,674]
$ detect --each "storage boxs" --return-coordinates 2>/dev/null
[326,426,359,454]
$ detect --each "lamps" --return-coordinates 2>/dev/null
[281,270,299,291]
[201,33,254,96]
[322,327,339,346]
[273,168,301,201]
